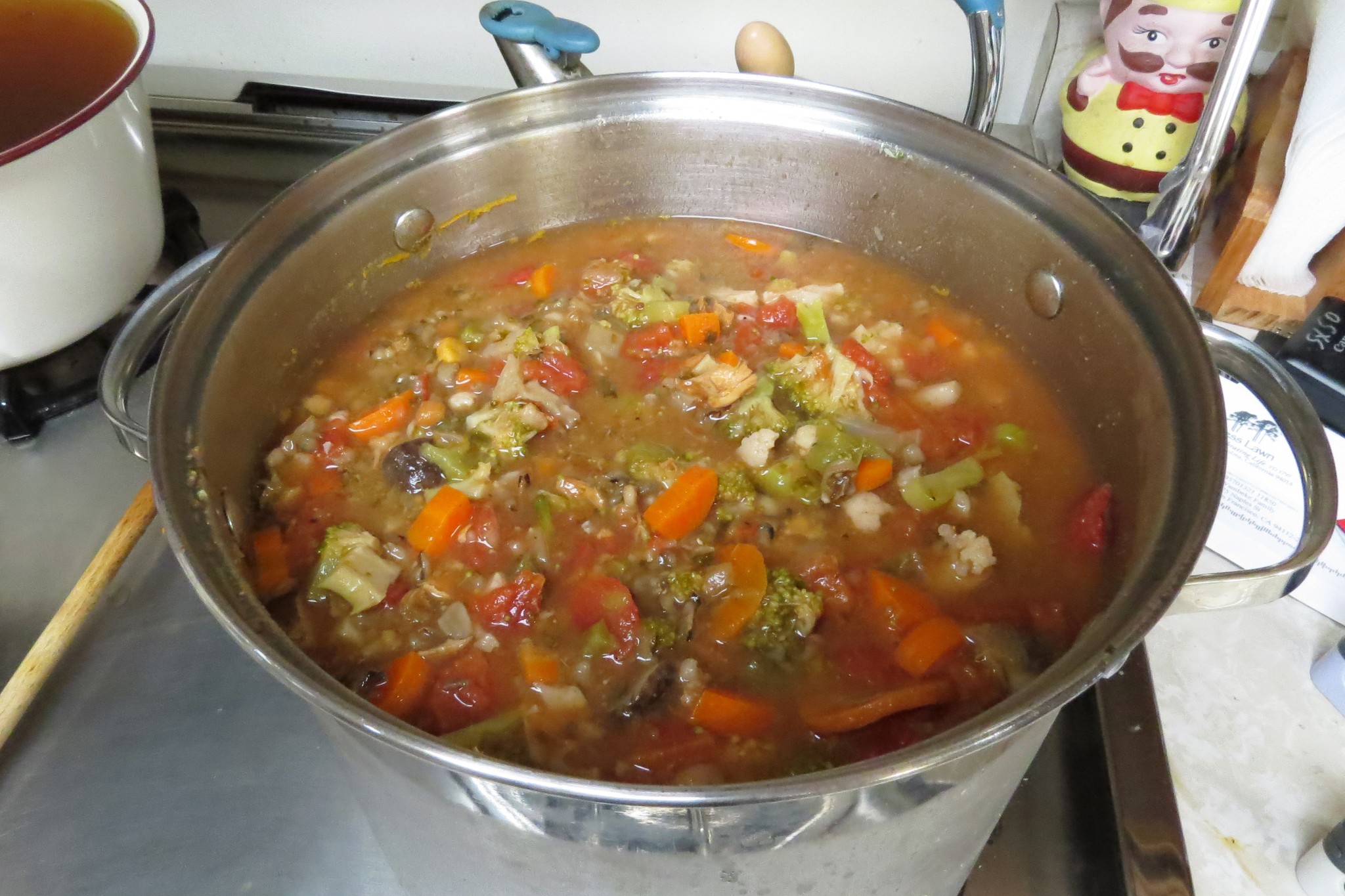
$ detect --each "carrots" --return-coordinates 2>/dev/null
[242,231,971,775]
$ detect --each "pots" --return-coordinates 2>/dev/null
[81,59,1338,868]
[0,0,167,374]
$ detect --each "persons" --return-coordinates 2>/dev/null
[1057,0,1249,202]
